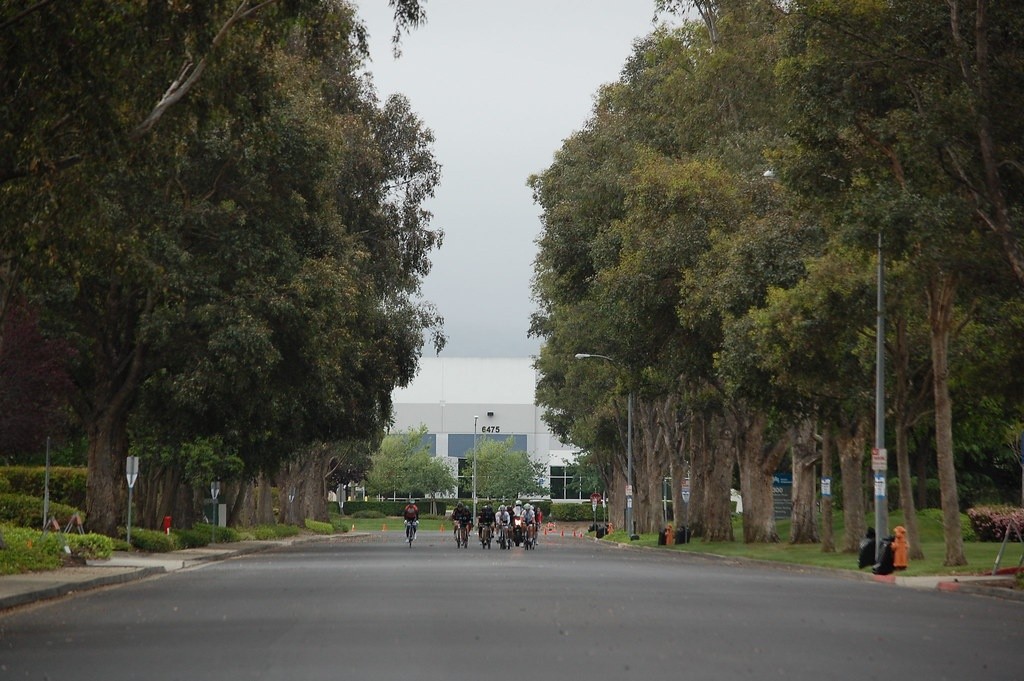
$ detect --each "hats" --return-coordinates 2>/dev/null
[408,496,543,518]
[866,527,875,538]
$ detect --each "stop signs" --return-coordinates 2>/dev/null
[591,493,601,504]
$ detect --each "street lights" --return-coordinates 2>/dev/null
[763,168,888,571]
[574,353,634,541]
[472,415,479,527]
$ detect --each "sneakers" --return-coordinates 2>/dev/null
[401,528,543,550]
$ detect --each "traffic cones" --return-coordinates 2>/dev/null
[440,524,444,532]
[578,530,583,538]
[352,524,355,532]
[562,529,564,536]
[382,523,386,532]
[544,528,546,535]
[573,530,576,537]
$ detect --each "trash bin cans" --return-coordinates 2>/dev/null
[202,502,227,526]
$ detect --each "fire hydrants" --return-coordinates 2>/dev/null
[664,524,673,545]
[607,522,614,535]
[890,526,911,569]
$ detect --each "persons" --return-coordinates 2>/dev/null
[404,498,419,543]
[451,499,542,548]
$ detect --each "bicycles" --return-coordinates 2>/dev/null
[452,516,541,550]
[405,521,418,548]
